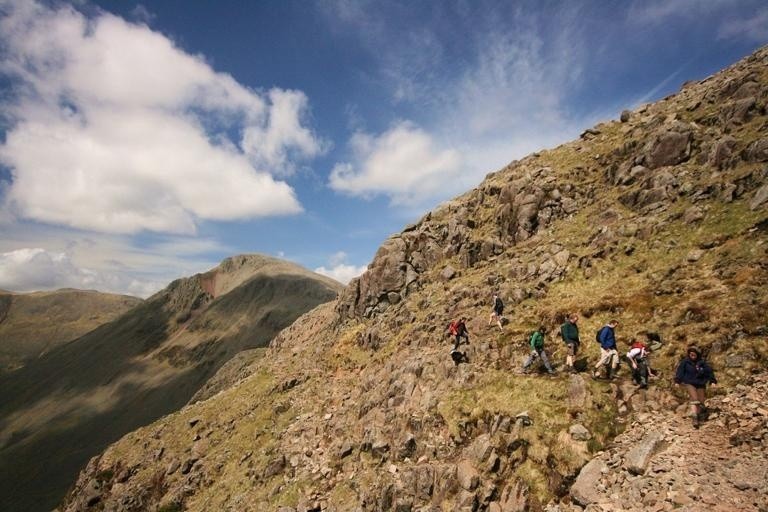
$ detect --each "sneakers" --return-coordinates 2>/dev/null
[692,415,700,429]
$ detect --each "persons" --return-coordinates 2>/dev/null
[450,317,470,352]
[561,314,580,374]
[487,293,503,330]
[523,325,554,373]
[590,320,619,380]
[675,348,717,427]
[626,346,655,387]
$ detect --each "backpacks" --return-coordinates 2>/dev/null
[632,342,646,349]
[596,327,605,343]
[496,298,503,308]
